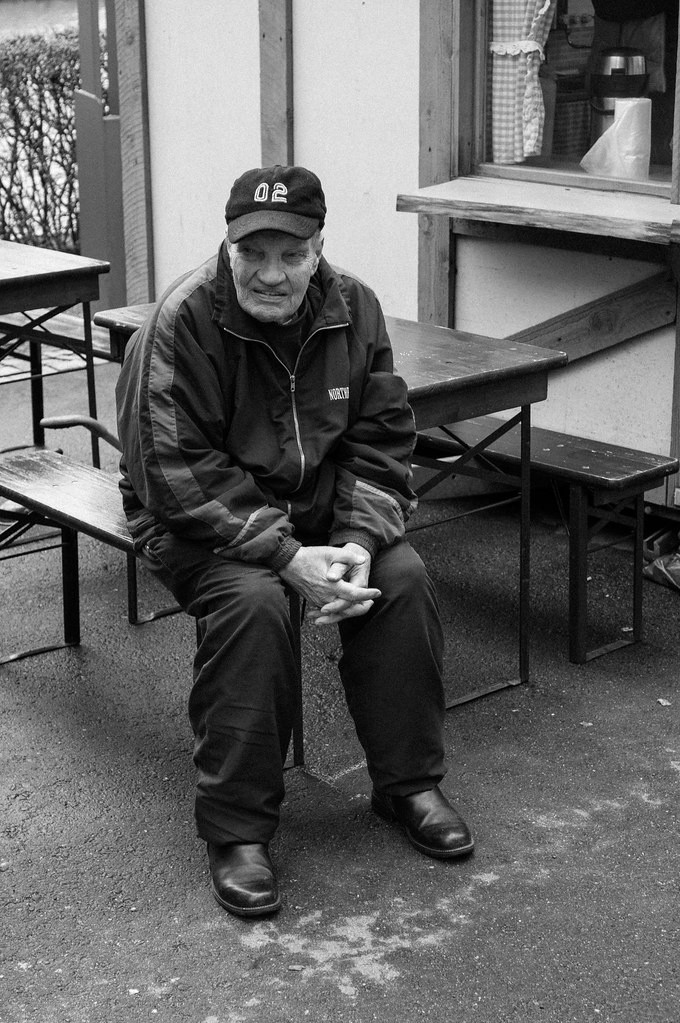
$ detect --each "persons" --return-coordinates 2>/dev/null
[113,162,481,920]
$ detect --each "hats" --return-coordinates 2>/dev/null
[224,162,330,246]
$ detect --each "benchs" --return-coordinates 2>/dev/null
[407,418,680,667]
[0,440,149,670]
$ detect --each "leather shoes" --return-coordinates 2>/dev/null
[369,778,475,858]
[198,838,281,914]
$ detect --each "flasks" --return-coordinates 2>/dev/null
[588,47,650,152]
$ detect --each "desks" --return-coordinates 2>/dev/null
[0,235,110,474]
[90,302,568,714]
